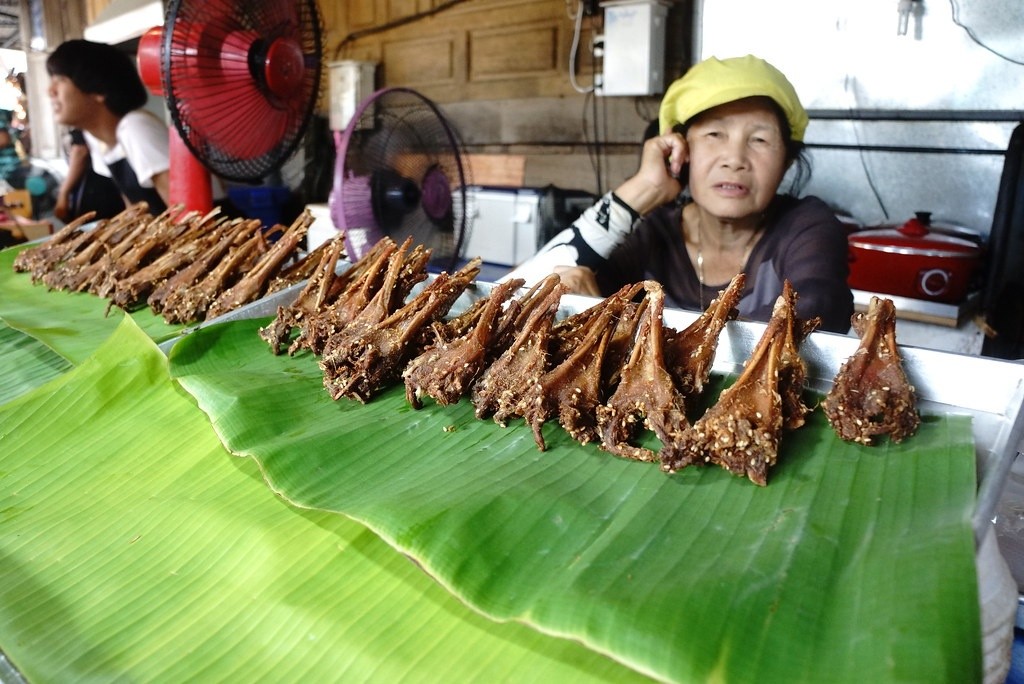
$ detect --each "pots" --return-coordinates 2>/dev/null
[845,207,985,308]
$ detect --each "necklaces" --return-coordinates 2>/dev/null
[696,207,765,312]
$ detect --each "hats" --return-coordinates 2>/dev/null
[658,55,810,167]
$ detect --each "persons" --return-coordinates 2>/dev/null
[497,53,855,340]
[47,39,171,213]
[53,124,125,227]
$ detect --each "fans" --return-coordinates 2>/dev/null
[332,79,477,279]
[135,0,331,236]
